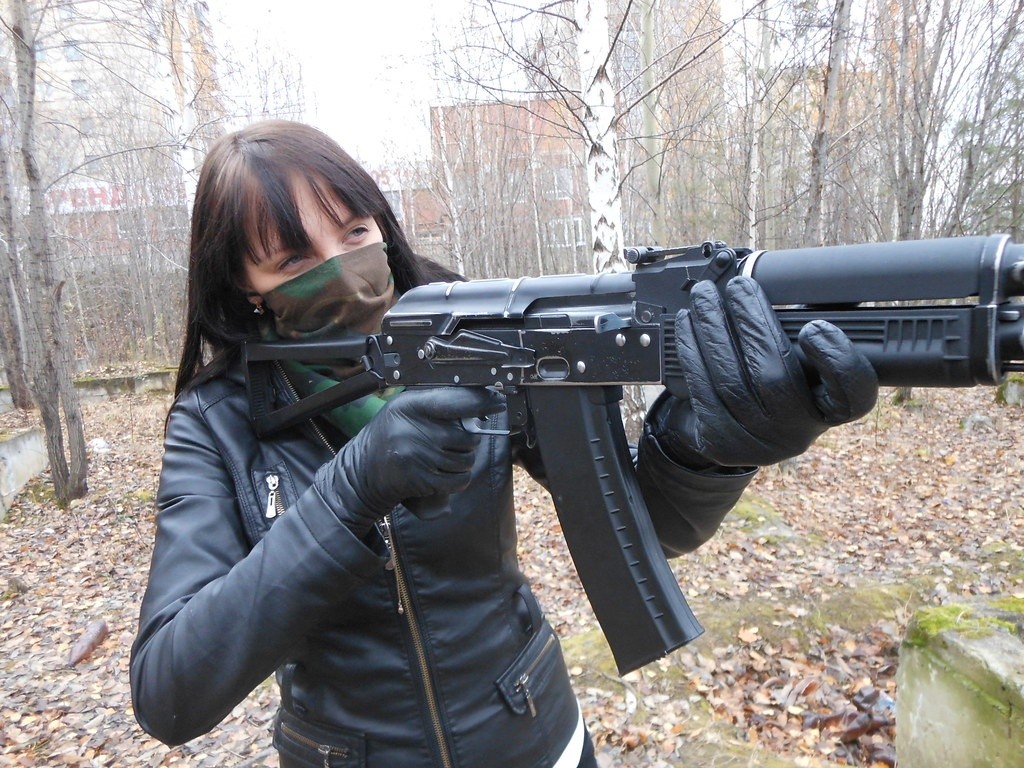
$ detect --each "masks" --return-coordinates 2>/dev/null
[258,243,394,339]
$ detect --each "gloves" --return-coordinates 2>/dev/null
[657,276,878,475]
[314,386,507,527]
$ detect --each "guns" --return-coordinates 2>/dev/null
[241,233,1024,677]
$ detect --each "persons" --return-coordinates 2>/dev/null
[128,122,876,768]
